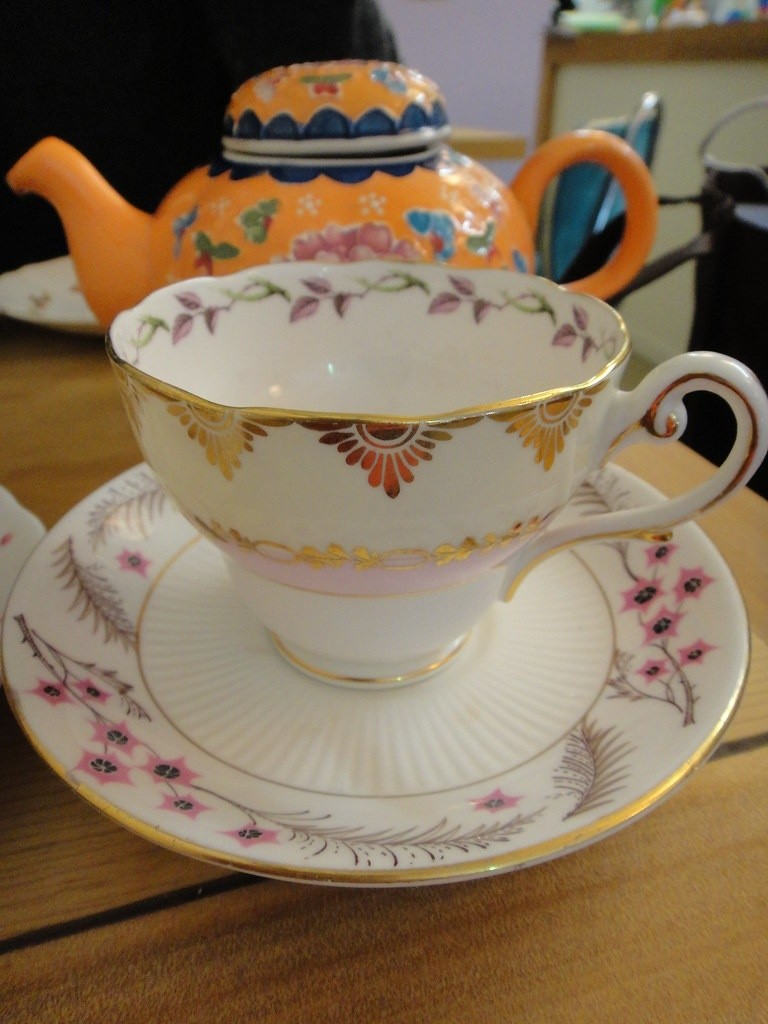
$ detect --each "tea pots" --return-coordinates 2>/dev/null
[6,58,658,340]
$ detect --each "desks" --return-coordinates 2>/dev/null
[1,323,766,1024]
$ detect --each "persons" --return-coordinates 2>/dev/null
[1,0,399,275]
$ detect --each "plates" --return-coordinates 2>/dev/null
[0,450,752,887]
[1,481,49,655]
[0,254,109,335]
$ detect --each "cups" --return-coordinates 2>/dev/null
[109,260,768,686]
